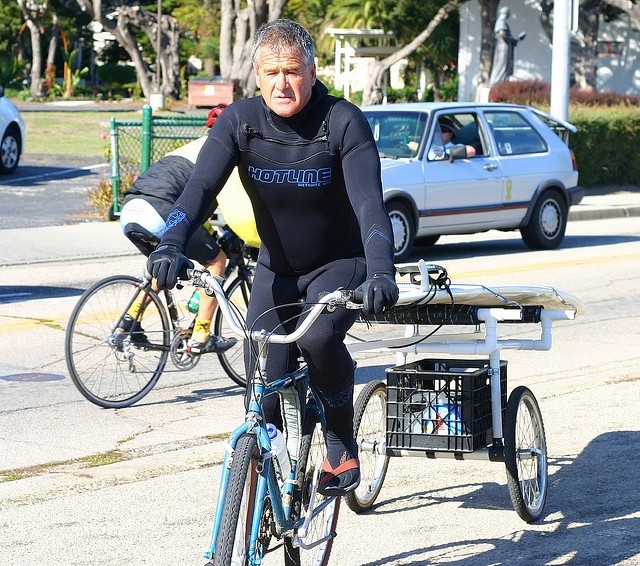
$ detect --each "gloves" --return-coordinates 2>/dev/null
[354,273,400,315]
[146,242,195,288]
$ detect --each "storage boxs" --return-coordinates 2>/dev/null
[385,357,508,453]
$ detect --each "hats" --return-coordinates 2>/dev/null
[435,118,454,132]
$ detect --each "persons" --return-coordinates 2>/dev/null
[487,5,527,91]
[146,19,399,498]
[110,105,245,357]
[408,116,475,161]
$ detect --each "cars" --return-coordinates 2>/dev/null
[357,103,582,263]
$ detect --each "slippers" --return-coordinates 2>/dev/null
[318,439,362,494]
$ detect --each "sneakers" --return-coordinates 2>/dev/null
[119,312,151,343]
[187,333,237,355]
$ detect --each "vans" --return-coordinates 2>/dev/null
[0,86,26,173]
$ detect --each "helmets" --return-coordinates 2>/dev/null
[205,102,230,126]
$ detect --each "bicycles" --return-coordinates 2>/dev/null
[142,254,367,566]
[66,215,263,408]
